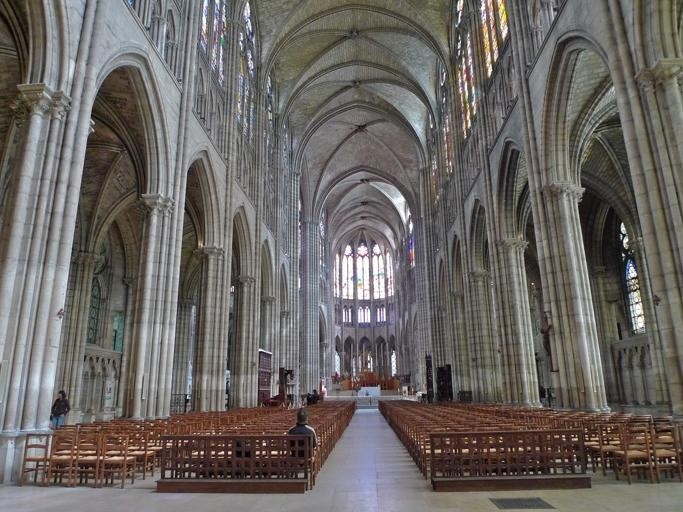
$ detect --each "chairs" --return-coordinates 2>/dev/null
[14,398,359,495]
[375,395,683,491]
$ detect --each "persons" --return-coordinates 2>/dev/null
[286,389,319,458]
[50,390,70,429]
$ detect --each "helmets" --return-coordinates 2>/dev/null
[297,408,308,424]
